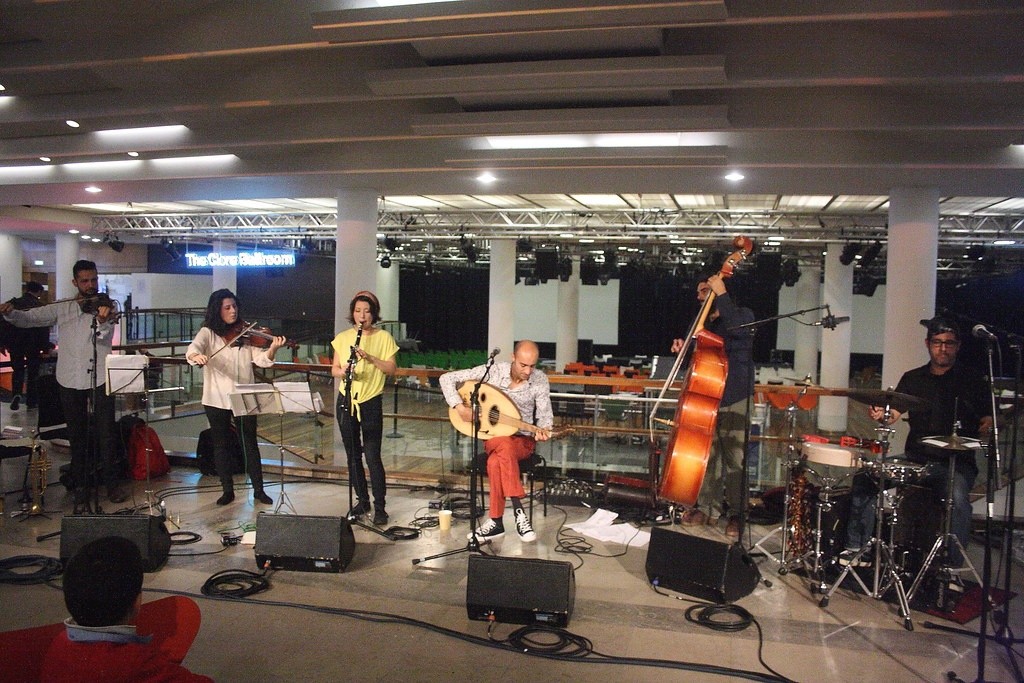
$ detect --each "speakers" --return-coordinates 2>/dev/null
[465,555,576,628]
[254,511,357,574]
[59,514,173,574]
[645,526,760,605]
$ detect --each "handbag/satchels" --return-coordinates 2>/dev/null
[38,409,69,440]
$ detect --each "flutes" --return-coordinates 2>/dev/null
[338,321,363,412]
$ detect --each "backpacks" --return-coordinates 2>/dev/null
[130,422,172,480]
[195,424,245,477]
[117,411,146,479]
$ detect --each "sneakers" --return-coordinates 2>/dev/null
[466,518,505,542]
[514,506,537,543]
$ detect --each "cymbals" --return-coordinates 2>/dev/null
[781,376,826,390]
[845,388,934,413]
[750,433,803,442]
[914,436,989,451]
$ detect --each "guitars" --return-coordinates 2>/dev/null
[448,380,576,440]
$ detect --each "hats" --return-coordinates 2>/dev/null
[926,316,960,337]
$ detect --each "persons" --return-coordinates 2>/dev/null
[329,292,400,525]
[869,315,996,595]
[439,340,552,540]
[670,275,754,537]
[0,259,216,683]
[185,289,285,505]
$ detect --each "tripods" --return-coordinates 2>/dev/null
[411,362,494,564]
[751,399,1005,632]
[342,356,397,540]
[241,390,315,514]
[109,366,184,529]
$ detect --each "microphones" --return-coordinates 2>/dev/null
[814,316,850,328]
[490,347,501,357]
[972,323,999,343]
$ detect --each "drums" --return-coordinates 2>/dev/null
[868,459,928,485]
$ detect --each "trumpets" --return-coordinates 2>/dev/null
[26,430,52,515]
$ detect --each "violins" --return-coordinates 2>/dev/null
[225,318,301,351]
[78,293,122,325]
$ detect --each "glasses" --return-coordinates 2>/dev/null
[931,338,959,348]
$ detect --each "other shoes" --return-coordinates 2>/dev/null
[254,489,273,505]
[10,394,21,410]
[374,509,388,525]
[106,480,125,503]
[73,500,89,514]
[351,499,371,514]
[217,491,235,505]
[27,405,38,411]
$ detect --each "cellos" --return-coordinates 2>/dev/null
[649,234,753,530]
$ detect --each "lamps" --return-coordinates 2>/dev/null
[97,232,895,295]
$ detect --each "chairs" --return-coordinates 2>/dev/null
[548,351,653,426]
[466,408,549,534]
